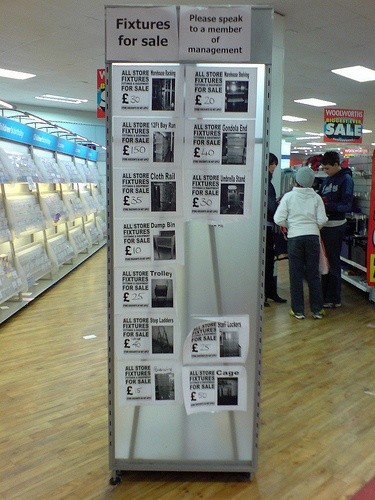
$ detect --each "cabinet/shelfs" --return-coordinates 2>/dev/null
[0,115,108,325]
[339,150,375,293]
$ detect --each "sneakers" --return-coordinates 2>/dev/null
[313,309,325,319]
[289,308,305,319]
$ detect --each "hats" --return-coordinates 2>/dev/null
[296,167,315,187]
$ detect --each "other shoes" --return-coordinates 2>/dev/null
[323,302,341,307]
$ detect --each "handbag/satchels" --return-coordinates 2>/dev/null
[319,235,330,275]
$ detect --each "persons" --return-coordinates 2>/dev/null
[274,166,328,320]
[264,152,287,307]
[316,150,354,308]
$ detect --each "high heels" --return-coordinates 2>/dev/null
[265,294,287,306]
